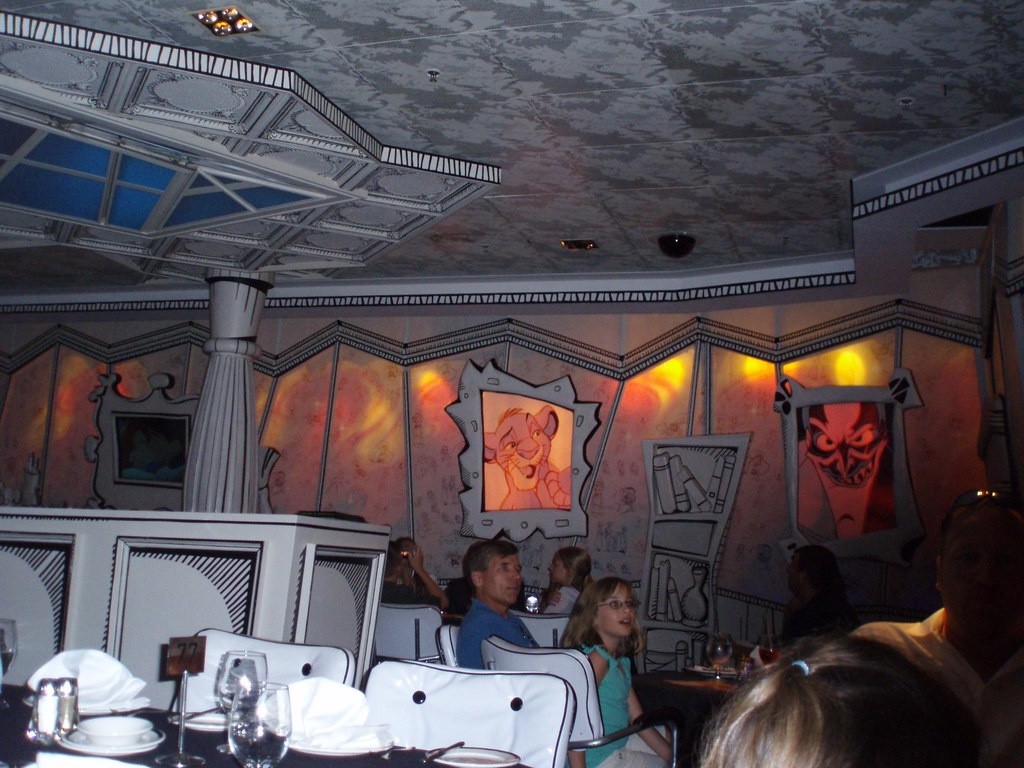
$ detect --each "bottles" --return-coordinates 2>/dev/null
[35,678,79,741]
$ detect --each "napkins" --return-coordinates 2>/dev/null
[0,682,532,768]
[255,674,396,753]
[28,649,148,706]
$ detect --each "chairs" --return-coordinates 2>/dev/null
[171,602,684,768]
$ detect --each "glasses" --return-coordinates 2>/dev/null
[950,485,1023,509]
[593,600,636,609]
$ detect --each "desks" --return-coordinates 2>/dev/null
[645,669,740,709]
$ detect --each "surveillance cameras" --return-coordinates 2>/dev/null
[657,234,695,258]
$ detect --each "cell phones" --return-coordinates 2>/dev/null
[400,547,416,560]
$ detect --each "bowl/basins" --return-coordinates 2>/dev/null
[78,716,153,748]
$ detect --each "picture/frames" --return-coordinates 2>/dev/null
[110,411,192,488]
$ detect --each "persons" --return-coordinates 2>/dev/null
[443,573,527,628]
[848,488,1024,768]
[697,630,984,768]
[542,545,594,615]
[380,537,457,627]
[559,576,681,768]
[779,545,861,649]
[456,539,541,669]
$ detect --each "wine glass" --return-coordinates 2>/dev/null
[216,651,268,752]
[0,619,18,709]
[706,634,732,684]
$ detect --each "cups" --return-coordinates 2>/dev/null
[759,634,781,665]
[228,681,292,768]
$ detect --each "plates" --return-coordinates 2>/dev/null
[285,741,393,758]
[425,747,521,768]
[169,711,228,732]
[684,664,738,678]
[23,695,111,716]
[57,730,167,756]
